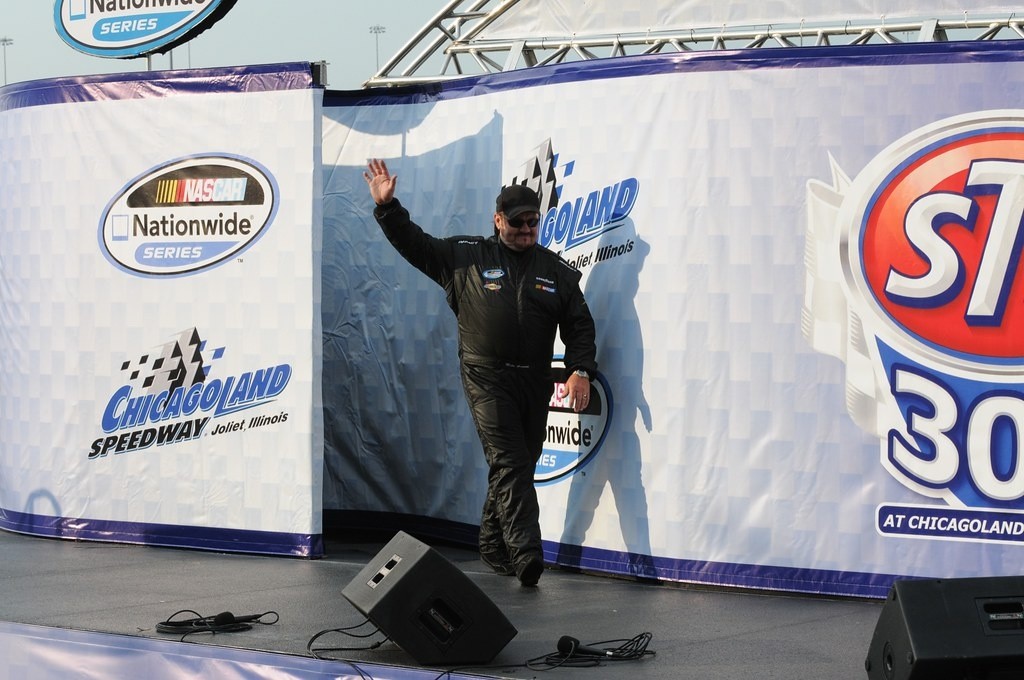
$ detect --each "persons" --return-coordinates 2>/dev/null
[363,158,599,585]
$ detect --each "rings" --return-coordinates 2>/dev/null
[582,395,588,400]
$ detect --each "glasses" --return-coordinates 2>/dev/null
[499,213,539,228]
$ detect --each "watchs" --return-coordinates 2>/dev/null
[572,370,589,379]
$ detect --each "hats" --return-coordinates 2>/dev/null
[496,185,539,218]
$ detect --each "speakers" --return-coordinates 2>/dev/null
[338,529,519,664]
[864,575,1022,679]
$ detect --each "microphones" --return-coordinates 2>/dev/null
[214,610,262,627]
[556,635,614,660]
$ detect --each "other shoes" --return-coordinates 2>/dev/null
[516,555,543,586]
[480,555,516,576]
